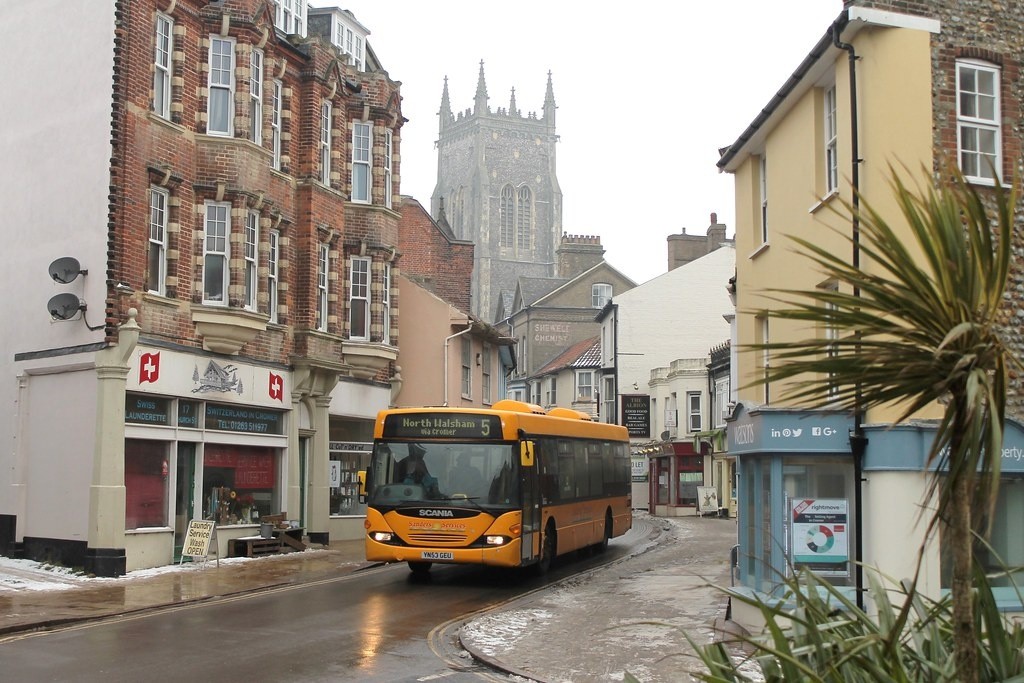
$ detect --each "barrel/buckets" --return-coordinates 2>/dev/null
[260,523,274,537]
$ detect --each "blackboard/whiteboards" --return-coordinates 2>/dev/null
[182,519,214,559]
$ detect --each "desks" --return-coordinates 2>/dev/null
[227,535,281,557]
[257,527,307,554]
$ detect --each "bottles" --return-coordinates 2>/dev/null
[332,465,336,481]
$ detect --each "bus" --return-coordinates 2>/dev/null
[356,399,633,573]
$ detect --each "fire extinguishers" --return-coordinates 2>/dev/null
[161,459,168,481]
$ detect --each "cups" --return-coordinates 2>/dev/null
[204,292,209,300]
